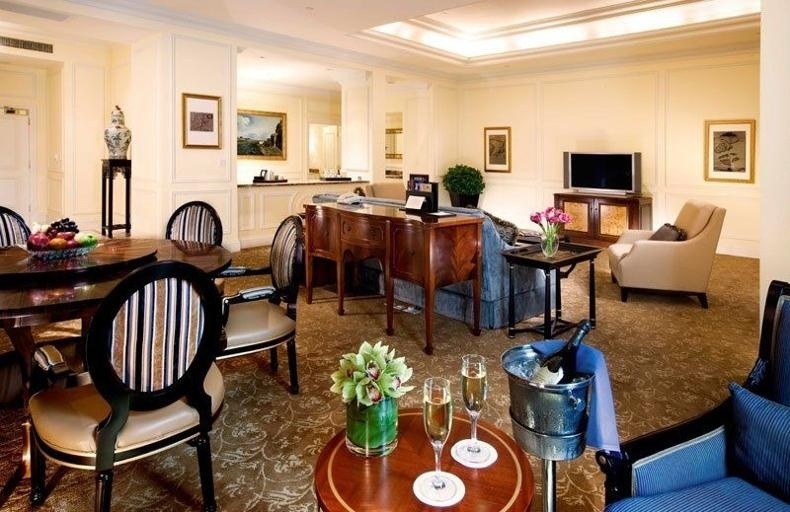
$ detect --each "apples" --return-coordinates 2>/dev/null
[57,232,96,249]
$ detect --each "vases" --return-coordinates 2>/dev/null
[539,232,559,257]
[343,400,400,457]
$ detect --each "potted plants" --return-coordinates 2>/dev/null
[442,164,486,208]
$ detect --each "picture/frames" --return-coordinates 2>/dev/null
[484,126,513,173]
[182,92,223,149]
[703,117,758,183]
[236,111,287,160]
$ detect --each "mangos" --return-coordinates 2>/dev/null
[50,238,66,249]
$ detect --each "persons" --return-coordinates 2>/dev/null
[713,132,744,172]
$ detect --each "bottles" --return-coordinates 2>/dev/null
[530,316,596,387]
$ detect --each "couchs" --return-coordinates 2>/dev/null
[595,278,789,512]
[311,193,558,328]
[608,198,728,309]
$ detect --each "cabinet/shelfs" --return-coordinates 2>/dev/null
[303,198,484,356]
[553,191,653,248]
[99,158,132,239]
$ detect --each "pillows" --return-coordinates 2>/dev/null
[727,382,790,502]
[485,210,519,245]
[648,223,686,241]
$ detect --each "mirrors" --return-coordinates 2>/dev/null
[384,127,404,159]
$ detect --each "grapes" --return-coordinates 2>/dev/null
[51,217,78,232]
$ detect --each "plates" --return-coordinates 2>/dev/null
[17,231,114,261]
[17,255,101,272]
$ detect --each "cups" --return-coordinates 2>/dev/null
[319,168,347,178]
[278,175,285,181]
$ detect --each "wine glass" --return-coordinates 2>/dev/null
[421,375,456,500]
[455,354,492,464]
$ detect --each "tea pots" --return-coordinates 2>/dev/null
[265,170,275,182]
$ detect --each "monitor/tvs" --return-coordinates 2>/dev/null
[564,152,642,198]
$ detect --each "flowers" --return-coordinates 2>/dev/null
[530,206,574,252]
[328,340,419,408]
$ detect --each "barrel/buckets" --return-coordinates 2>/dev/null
[500,343,596,461]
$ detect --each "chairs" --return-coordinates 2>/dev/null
[27,258,227,512]
[213,215,305,395]
[165,200,224,244]
[0,204,35,249]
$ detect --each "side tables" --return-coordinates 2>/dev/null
[501,242,601,340]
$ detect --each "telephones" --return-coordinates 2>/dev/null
[337,193,362,205]
